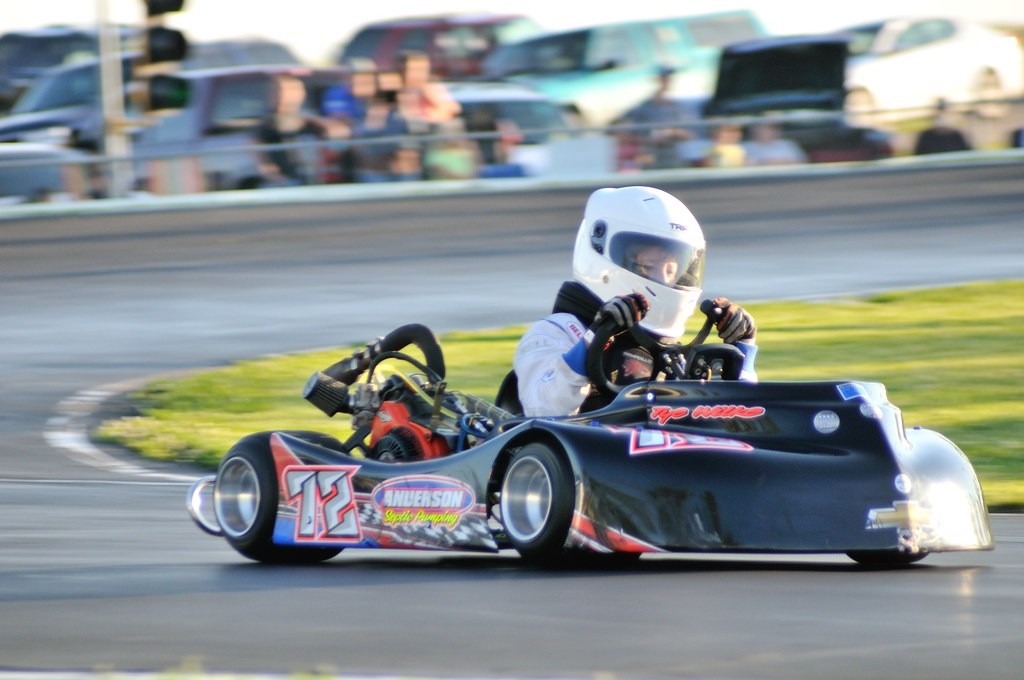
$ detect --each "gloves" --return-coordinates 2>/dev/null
[593,292,650,345]
[714,298,756,347]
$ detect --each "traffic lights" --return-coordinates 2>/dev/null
[134,0,194,110]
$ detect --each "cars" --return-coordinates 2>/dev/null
[1,9,1024,204]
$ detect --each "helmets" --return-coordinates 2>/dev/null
[572,186,705,336]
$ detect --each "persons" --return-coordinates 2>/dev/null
[606,66,811,170]
[534,104,616,175]
[257,58,481,189]
[493,186,759,419]
[913,97,969,154]
[811,89,893,161]
